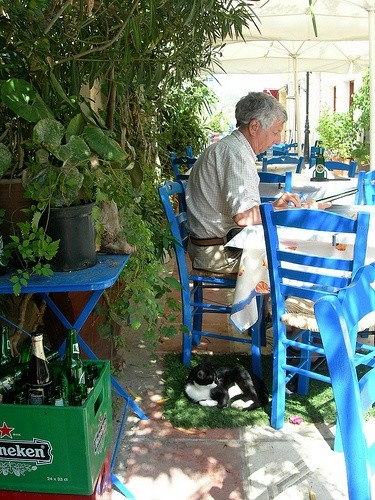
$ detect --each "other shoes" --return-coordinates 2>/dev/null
[288,346,328,371]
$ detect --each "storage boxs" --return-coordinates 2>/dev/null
[0,360,113,500]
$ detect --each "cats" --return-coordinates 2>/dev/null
[183,360,268,412]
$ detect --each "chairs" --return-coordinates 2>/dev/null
[158,142,375,500]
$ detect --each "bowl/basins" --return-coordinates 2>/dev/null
[333,169,349,178]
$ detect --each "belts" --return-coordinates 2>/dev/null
[190,236,224,246]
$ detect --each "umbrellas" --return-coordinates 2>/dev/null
[157,0,375,162]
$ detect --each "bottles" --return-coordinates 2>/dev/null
[0,363,98,407]
[0,349,60,402]
[316,147,324,178]
[28,334,52,405]
[60,328,83,406]
[0,325,12,377]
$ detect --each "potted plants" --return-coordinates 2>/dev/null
[0,70,191,378]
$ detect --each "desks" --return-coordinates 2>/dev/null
[0,251,148,500]
[258,171,358,205]
[222,202,375,394]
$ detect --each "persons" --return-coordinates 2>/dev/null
[185,92,320,362]
